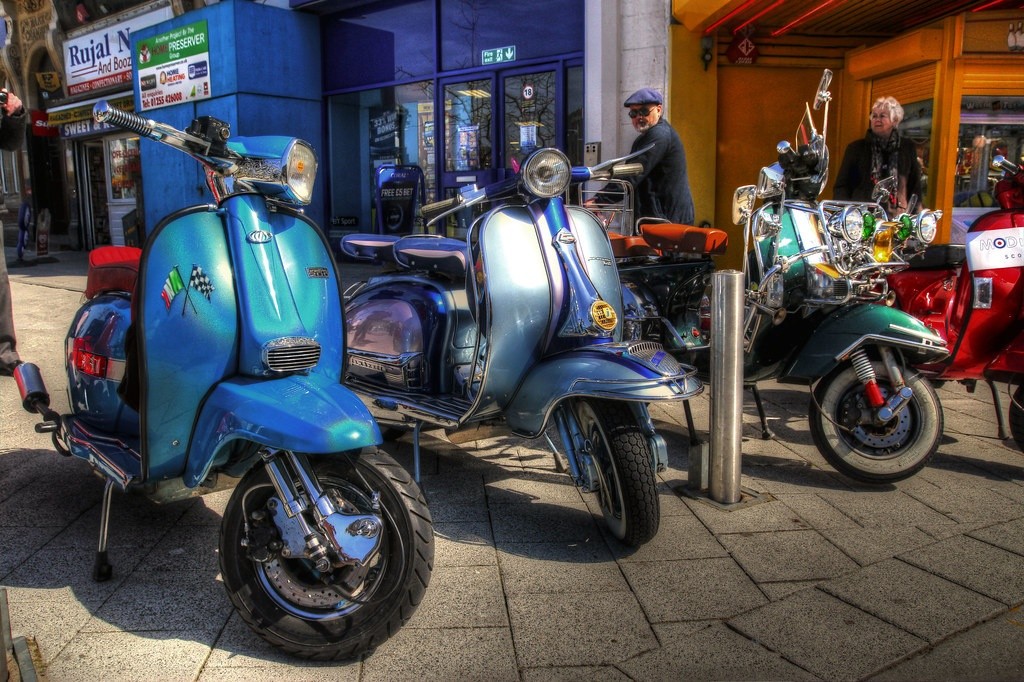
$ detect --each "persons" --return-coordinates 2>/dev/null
[0,88,28,379]
[582,88,696,234]
[832,95,923,217]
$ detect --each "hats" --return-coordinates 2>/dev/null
[624,87,663,107]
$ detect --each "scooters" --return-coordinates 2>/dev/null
[595,64,949,486]
[14,100,438,668]
[857,150,1024,458]
[330,142,704,548]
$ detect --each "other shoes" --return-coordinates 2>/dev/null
[0,360,39,376]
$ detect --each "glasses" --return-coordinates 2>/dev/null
[870,113,889,120]
[628,106,657,118]
